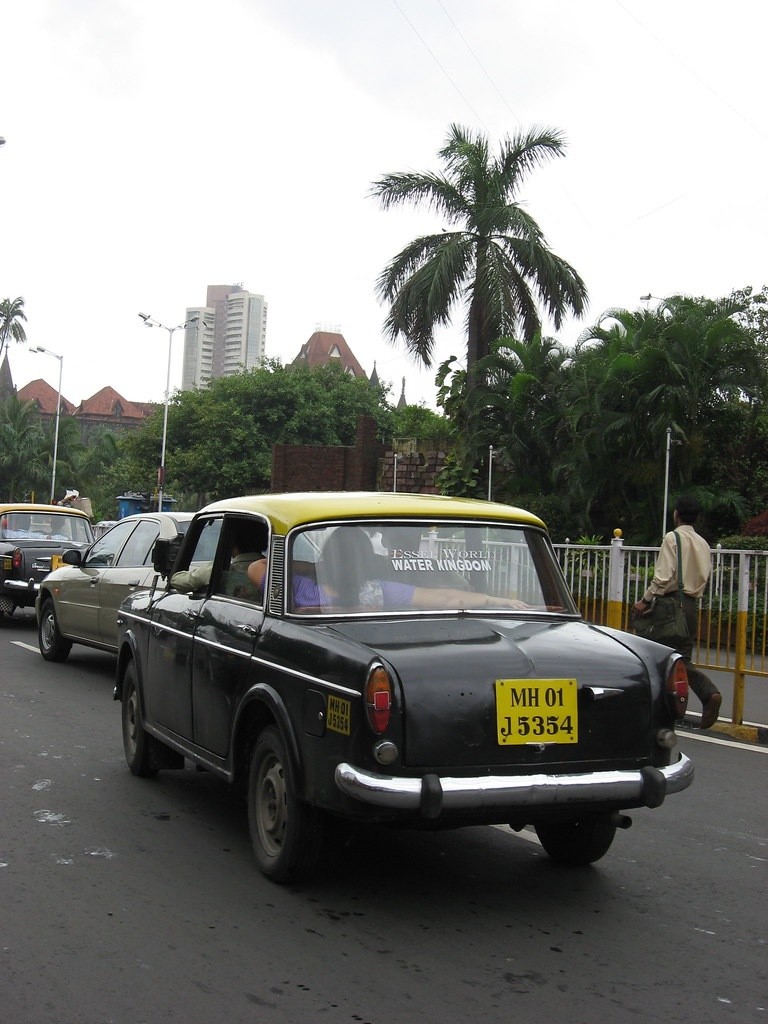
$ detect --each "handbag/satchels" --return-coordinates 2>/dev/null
[636,589,692,649]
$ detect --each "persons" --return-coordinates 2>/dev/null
[631,495,722,730]
[2,515,71,541]
[48,516,99,548]
[169,518,268,603]
[247,527,528,610]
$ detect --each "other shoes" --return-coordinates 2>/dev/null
[699,693,722,729]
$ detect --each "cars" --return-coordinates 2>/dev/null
[0,503,96,618]
[34,512,222,662]
[113,491,697,887]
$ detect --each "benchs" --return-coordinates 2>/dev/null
[298,606,566,616]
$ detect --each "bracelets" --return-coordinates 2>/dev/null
[642,597,649,605]
[485,595,488,607]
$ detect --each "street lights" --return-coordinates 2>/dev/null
[28,347,63,505]
[137,312,201,513]
